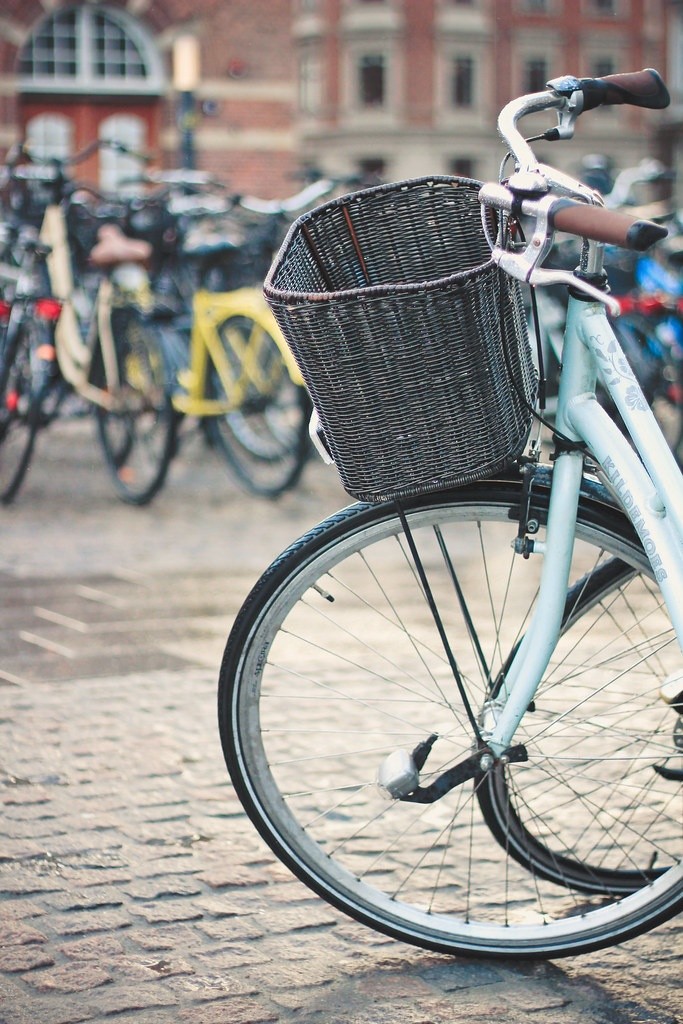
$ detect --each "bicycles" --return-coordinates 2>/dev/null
[216,62,682,962]
[0,141,682,507]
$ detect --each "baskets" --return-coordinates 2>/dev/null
[261,174,540,502]
